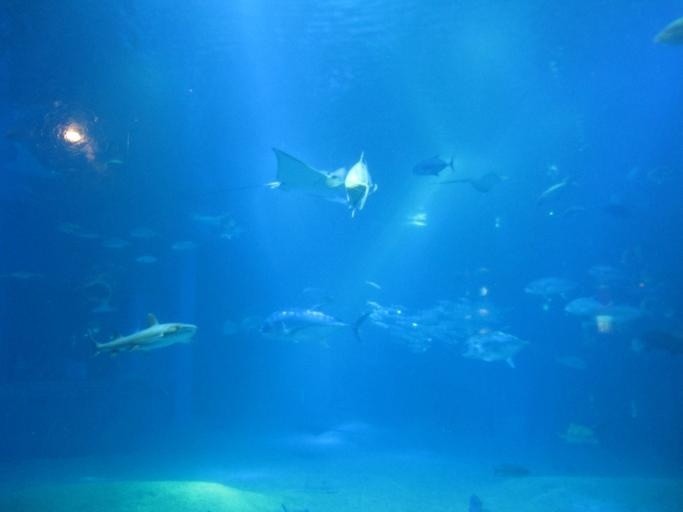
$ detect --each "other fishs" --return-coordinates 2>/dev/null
[651,17,683,44]
